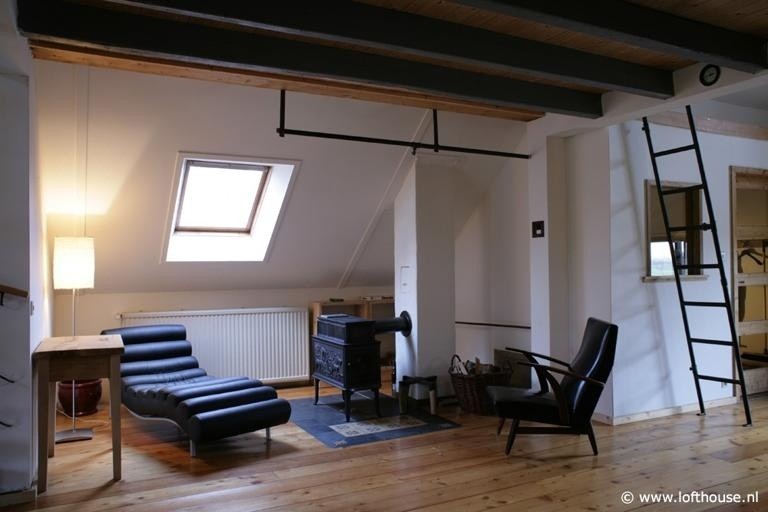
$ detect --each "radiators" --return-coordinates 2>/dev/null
[114,304,312,389]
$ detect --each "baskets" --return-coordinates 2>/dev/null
[450,355,512,414]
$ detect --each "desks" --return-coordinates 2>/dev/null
[31,334,125,494]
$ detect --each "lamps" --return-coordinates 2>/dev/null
[53,237,94,444]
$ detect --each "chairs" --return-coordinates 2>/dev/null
[484,317,618,456]
[101,325,292,457]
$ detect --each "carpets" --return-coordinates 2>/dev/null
[286,390,463,449]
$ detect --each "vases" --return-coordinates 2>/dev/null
[57,379,101,418]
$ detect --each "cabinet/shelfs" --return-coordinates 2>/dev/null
[308,296,396,387]
